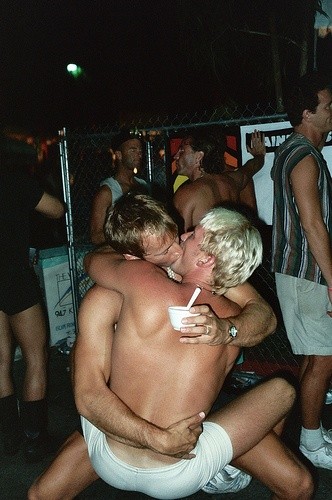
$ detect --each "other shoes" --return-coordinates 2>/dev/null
[299,428,332,471]
[324,388,332,404]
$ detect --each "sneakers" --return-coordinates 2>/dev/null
[202,465,252,494]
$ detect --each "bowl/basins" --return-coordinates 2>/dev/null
[168,306,201,331]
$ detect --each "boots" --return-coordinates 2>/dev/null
[0,394,23,455]
[19,393,48,458]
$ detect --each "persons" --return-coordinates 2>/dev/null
[0,110,316,500]
[270,73,332,471]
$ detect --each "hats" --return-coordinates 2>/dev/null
[111,133,145,152]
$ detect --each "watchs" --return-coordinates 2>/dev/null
[225,319,237,345]
